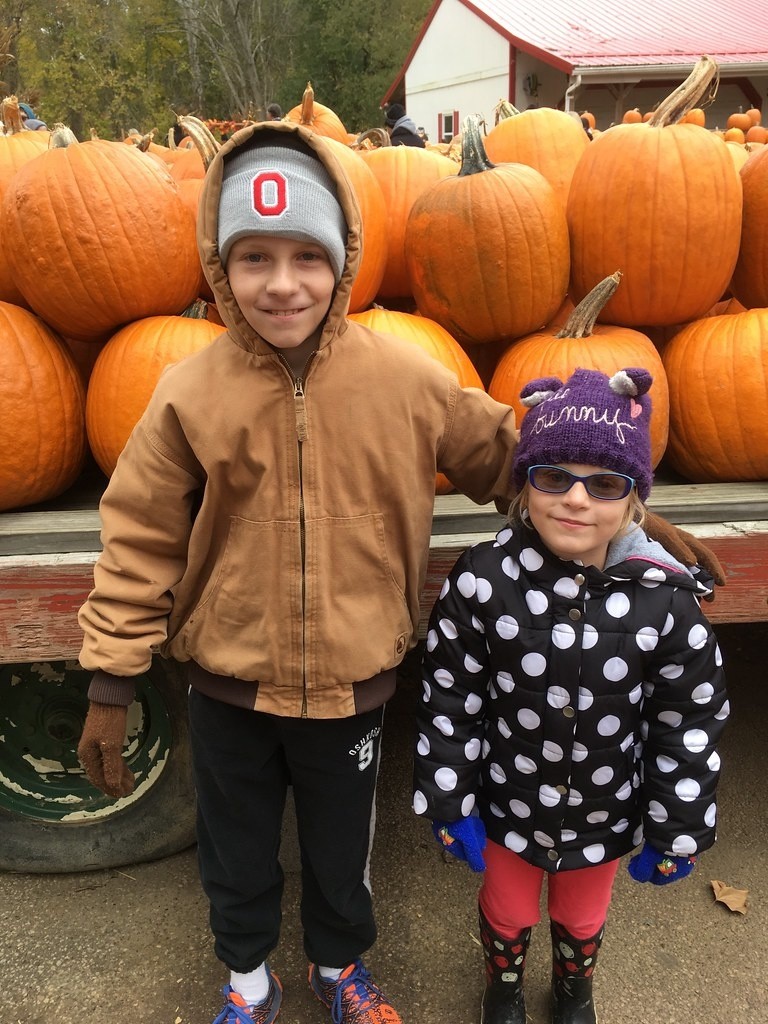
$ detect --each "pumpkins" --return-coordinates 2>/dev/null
[278,52,767,495]
[0,95,223,513]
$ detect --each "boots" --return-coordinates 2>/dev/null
[548,917,605,1024]
[477,902,531,1024]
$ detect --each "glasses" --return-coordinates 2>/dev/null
[526,465,637,500]
[22,116,28,120]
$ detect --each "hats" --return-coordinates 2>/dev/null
[18,102,35,119]
[26,119,49,131]
[511,367,653,503]
[217,146,348,287]
[385,104,407,121]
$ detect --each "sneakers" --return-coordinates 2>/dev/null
[211,961,283,1024]
[308,960,404,1024]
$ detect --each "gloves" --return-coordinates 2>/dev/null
[627,843,698,886]
[632,504,726,602]
[77,701,135,798]
[431,815,488,872]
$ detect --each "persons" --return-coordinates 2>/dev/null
[268,104,282,122]
[164,124,185,147]
[18,103,47,131]
[582,118,593,139]
[79,123,726,1024]
[415,367,731,1024]
[386,105,426,148]
